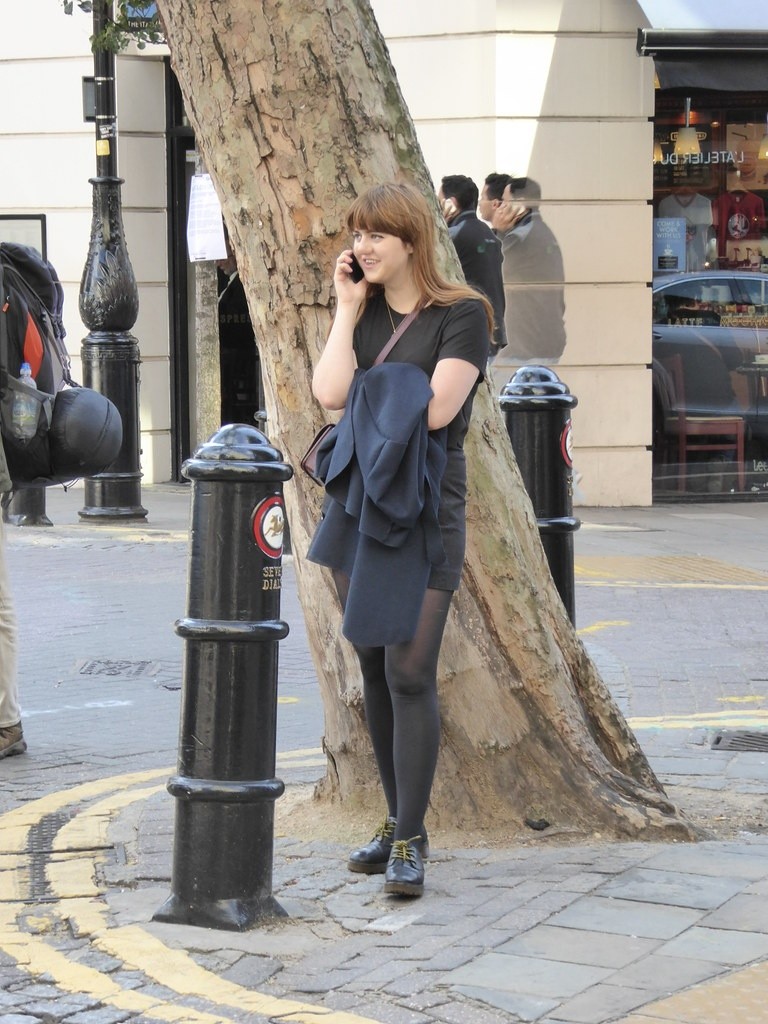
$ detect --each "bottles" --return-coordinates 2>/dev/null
[12,363,36,439]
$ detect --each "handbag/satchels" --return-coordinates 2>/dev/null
[300,423,337,489]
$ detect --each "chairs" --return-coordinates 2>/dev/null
[654,354,744,491]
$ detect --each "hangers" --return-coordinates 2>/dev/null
[675,181,694,194]
[728,172,748,194]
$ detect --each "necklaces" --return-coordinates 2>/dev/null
[384,294,420,333]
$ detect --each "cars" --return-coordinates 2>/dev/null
[652,271,768,464]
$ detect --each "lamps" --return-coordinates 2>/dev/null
[758,111,768,159]
[653,137,663,162]
[674,96,700,154]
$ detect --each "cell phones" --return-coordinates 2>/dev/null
[511,203,526,216]
[442,198,457,215]
[346,253,365,284]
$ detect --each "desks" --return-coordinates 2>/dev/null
[736,363,768,492]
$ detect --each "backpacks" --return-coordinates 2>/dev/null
[1,243,123,487]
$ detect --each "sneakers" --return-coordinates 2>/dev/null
[0,722,26,761]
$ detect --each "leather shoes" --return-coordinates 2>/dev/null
[383,836,428,896]
[349,817,427,873]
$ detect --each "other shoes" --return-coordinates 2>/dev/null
[34,514,54,527]
[6,508,34,527]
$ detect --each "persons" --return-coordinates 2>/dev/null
[478,171,514,238]
[218,240,253,423]
[654,281,745,491]
[492,177,566,407]
[0,240,28,763]
[307,180,493,898]
[437,174,507,372]
